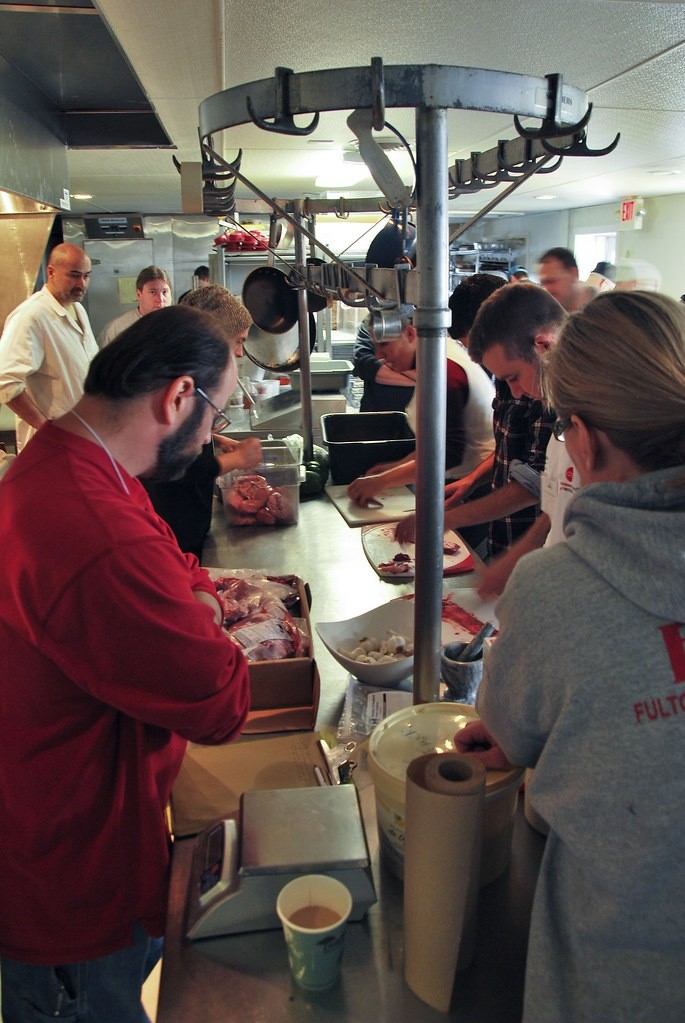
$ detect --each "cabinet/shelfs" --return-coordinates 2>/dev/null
[449,248,512,292]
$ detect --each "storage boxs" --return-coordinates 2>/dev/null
[287,360,355,392]
[214,439,306,524]
[319,411,417,486]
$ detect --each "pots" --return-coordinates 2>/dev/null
[242,258,328,371]
[364,208,408,268]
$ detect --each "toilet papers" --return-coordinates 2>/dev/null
[403,752,488,1018]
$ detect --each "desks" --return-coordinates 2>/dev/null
[156,496,549,1023]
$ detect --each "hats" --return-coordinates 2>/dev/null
[507,265,529,282]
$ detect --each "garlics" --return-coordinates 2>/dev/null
[337,630,414,663]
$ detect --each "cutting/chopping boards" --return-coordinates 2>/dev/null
[325,481,416,524]
[362,520,475,577]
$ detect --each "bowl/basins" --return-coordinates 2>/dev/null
[315,600,418,687]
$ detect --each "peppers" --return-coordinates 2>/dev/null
[301,461,329,493]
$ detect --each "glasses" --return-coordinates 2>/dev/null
[191,384,231,435]
[551,416,574,442]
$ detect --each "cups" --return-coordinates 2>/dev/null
[229,405,245,425]
[214,228,269,251]
[277,875,353,992]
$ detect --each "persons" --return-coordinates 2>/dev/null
[178,265,210,305]
[0,305,249,1022]
[453,290,685,1023]
[0,243,99,455]
[99,266,172,348]
[352,249,623,606]
[134,284,266,562]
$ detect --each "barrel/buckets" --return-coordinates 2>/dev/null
[367,702,520,895]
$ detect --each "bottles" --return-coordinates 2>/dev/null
[224,366,290,409]
